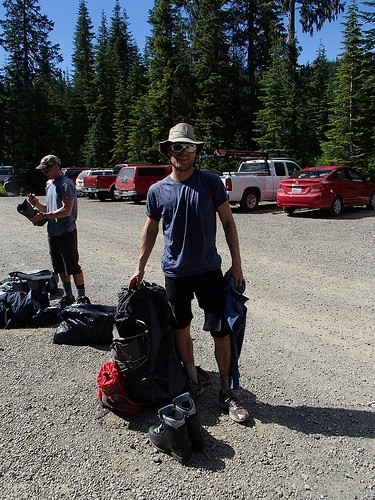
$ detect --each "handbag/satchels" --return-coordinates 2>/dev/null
[0,270,63,330]
[52,296,117,346]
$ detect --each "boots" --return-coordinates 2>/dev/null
[148,392,204,465]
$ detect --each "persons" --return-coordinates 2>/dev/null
[128,123,251,423]
[27,155,90,306]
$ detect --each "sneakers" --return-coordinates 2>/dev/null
[180,379,205,400]
[216,389,251,422]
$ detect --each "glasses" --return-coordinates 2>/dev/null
[41,166,52,174]
[167,144,197,153]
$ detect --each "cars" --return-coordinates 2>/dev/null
[277,167,375,217]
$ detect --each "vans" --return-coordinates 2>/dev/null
[62,164,172,203]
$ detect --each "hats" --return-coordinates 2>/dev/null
[159,123,206,161]
[35,154,61,169]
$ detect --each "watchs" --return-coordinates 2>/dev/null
[43,213,47,219]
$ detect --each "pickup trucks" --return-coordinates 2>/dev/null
[218,160,302,213]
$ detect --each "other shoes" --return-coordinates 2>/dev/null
[58,294,75,306]
[77,295,91,305]
